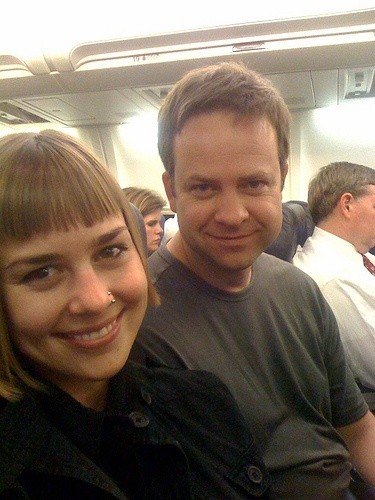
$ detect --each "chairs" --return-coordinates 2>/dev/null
[265,200,315,264]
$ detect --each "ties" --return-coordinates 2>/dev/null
[361,253,375,277]
